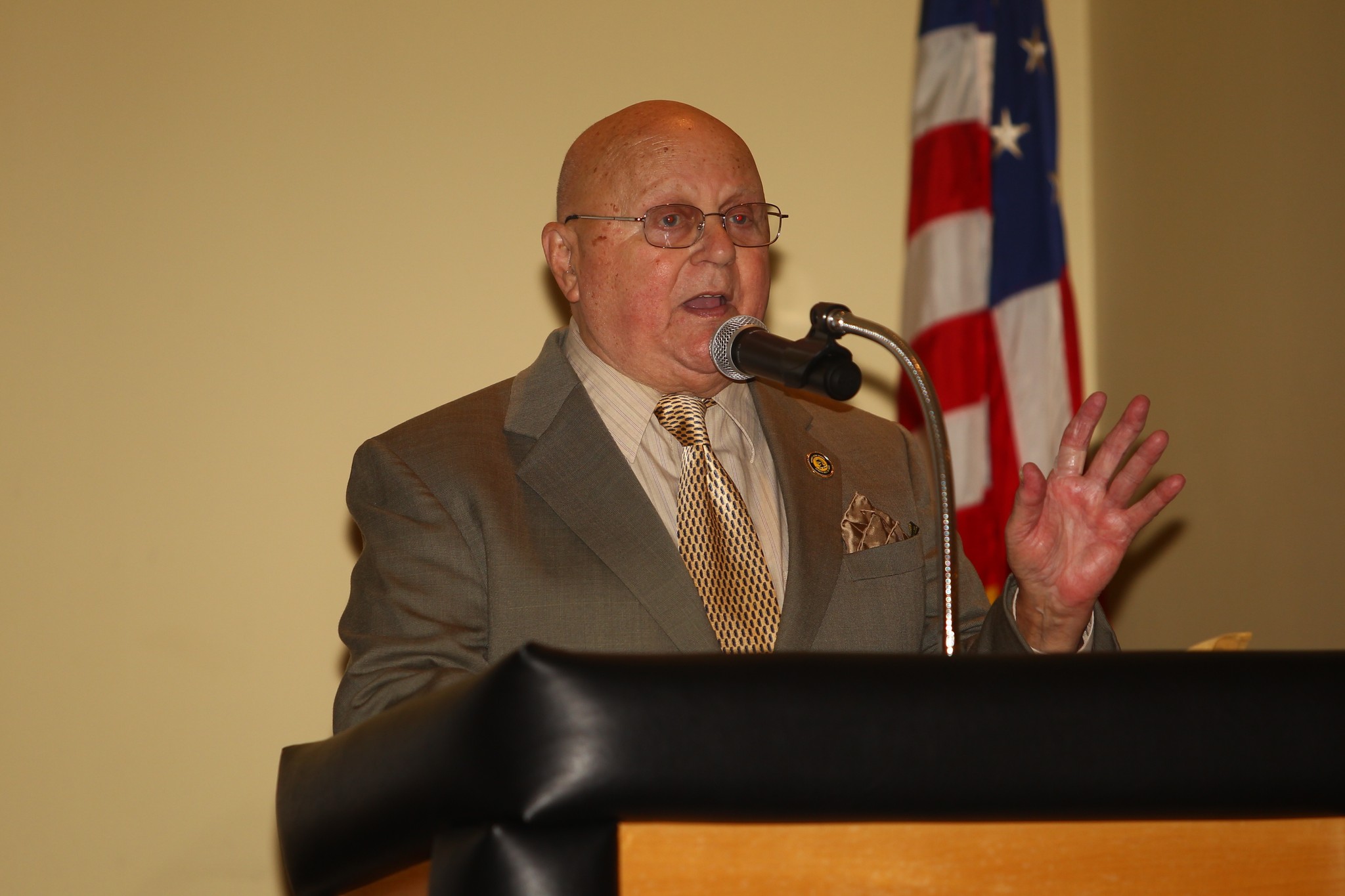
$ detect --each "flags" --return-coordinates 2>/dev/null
[892,0,1091,611]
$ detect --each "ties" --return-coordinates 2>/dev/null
[653,395,782,654]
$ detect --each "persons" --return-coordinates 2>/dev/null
[326,95,1193,740]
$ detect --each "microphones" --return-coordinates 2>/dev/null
[709,313,862,403]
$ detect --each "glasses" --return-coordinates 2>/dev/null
[564,204,789,249]
